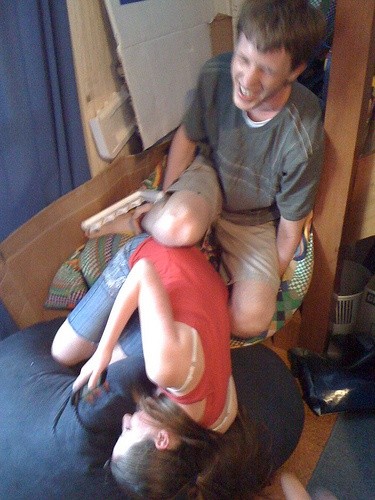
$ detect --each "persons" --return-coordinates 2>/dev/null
[50,232,275,499]
[84,0,325,340]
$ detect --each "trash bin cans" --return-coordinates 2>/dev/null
[327,259,372,334]
